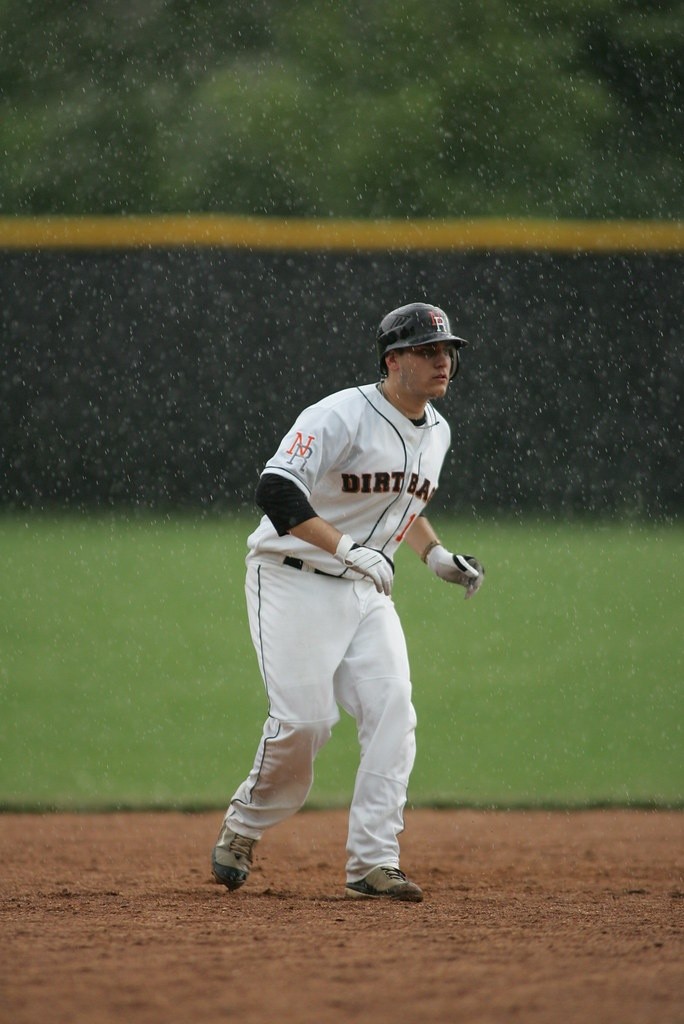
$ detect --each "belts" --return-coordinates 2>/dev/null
[283,555,343,578]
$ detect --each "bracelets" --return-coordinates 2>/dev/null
[422,541,439,564]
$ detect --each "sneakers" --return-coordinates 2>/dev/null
[345,866,424,902]
[212,823,255,889]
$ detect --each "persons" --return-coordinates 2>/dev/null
[213,302,483,902]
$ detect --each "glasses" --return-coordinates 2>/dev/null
[404,344,456,359]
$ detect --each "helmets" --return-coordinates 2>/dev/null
[377,302,469,381]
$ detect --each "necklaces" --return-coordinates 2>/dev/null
[380,379,439,429]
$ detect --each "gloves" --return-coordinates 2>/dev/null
[332,535,396,596]
[428,546,485,600]
